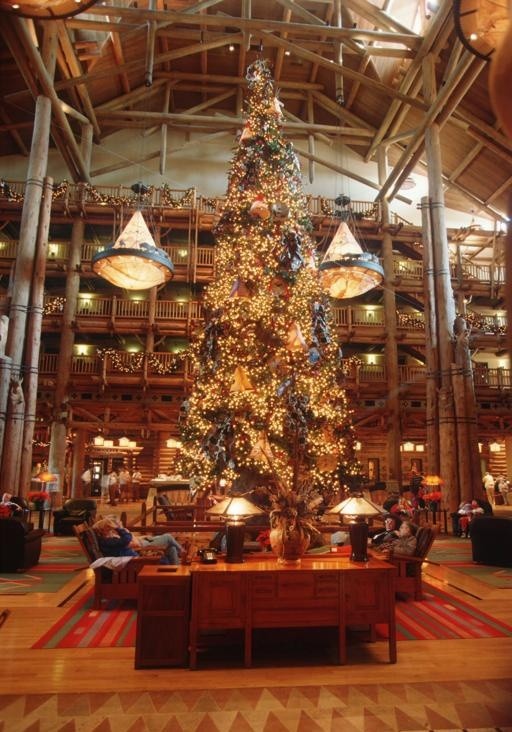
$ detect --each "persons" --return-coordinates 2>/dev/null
[410,469,425,495]
[130,465,143,502]
[105,468,118,507]
[458,498,485,538]
[495,473,511,505]
[94,517,188,565]
[368,515,401,546]
[380,521,418,563]
[103,513,162,548]
[117,466,132,503]
[80,467,93,497]
[481,471,496,506]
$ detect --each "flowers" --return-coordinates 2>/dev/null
[27,491,49,502]
[423,491,445,501]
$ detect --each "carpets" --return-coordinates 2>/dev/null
[24,580,509,650]
[0,573,94,593]
[423,542,512,589]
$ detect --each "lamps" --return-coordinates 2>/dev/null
[90,85,175,291]
[328,491,386,563]
[205,496,264,563]
[319,104,386,300]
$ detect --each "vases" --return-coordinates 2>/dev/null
[430,501,438,512]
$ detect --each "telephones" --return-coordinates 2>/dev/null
[197,547,219,563]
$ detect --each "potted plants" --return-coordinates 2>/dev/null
[258,445,325,565]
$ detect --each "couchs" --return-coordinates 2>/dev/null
[450,498,512,568]
[0,495,96,572]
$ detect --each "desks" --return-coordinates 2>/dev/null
[189,557,402,670]
[412,507,448,537]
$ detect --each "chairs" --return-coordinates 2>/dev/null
[73,514,169,604]
[157,494,195,521]
[367,521,436,601]
